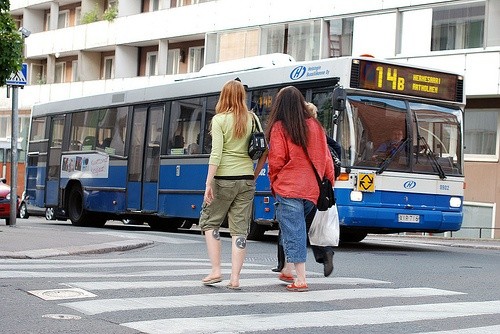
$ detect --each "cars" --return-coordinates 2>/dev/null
[0,178,21,225]
[19,190,70,221]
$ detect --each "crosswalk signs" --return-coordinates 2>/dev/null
[6,64,28,85]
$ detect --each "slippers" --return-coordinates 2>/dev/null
[202,276,222,284]
[225,283,242,290]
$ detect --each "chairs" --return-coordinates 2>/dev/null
[54,136,112,151]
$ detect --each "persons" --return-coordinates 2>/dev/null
[198,80,269,289]
[265,86,335,294]
[374,130,430,156]
[272,102,342,276]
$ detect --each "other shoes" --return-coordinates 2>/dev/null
[286,282,309,292]
[272,261,284,272]
[322,250,335,278]
[279,273,295,283]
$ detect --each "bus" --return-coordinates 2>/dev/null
[22,53,468,244]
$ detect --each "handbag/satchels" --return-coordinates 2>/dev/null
[307,202,340,248]
[248,132,267,161]
[316,176,337,211]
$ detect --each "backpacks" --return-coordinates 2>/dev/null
[327,144,342,181]
